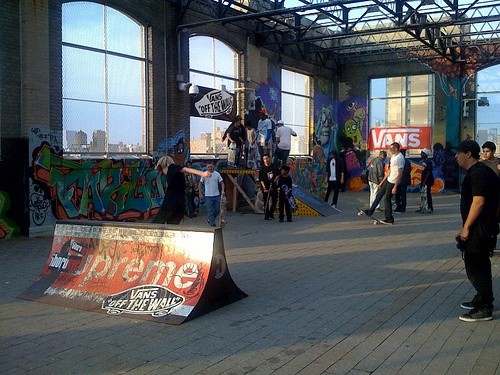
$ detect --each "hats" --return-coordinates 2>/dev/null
[450,140,480,153]
[259,109,266,114]
[275,120,284,125]
[330,151,337,156]
[421,148,430,156]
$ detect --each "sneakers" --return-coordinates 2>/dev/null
[269,214,275,219]
[394,207,405,212]
[458,307,494,322]
[427,208,433,214]
[460,296,495,310]
[380,217,394,225]
[287,219,292,222]
[279,219,284,222]
[206,219,216,227]
[264,217,272,220]
[415,207,422,212]
[364,210,373,216]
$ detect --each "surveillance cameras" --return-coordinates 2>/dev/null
[189,84,199,97]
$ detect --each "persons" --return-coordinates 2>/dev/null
[198,161,225,227]
[277,166,292,222]
[365,154,375,191]
[415,148,434,214]
[324,150,345,208]
[227,116,245,168]
[394,149,411,212]
[368,151,387,211]
[259,154,280,220]
[455,140,500,322]
[479,141,500,253]
[274,120,297,168]
[184,162,198,218]
[256,109,272,157]
[265,115,275,158]
[244,121,257,145]
[151,156,213,225]
[221,115,246,143]
[360,142,405,223]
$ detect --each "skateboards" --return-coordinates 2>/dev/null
[247,144,255,168]
[227,142,236,169]
[356,208,380,225]
[282,184,297,212]
[239,142,246,169]
[219,195,228,228]
[420,184,429,213]
[265,183,273,215]
